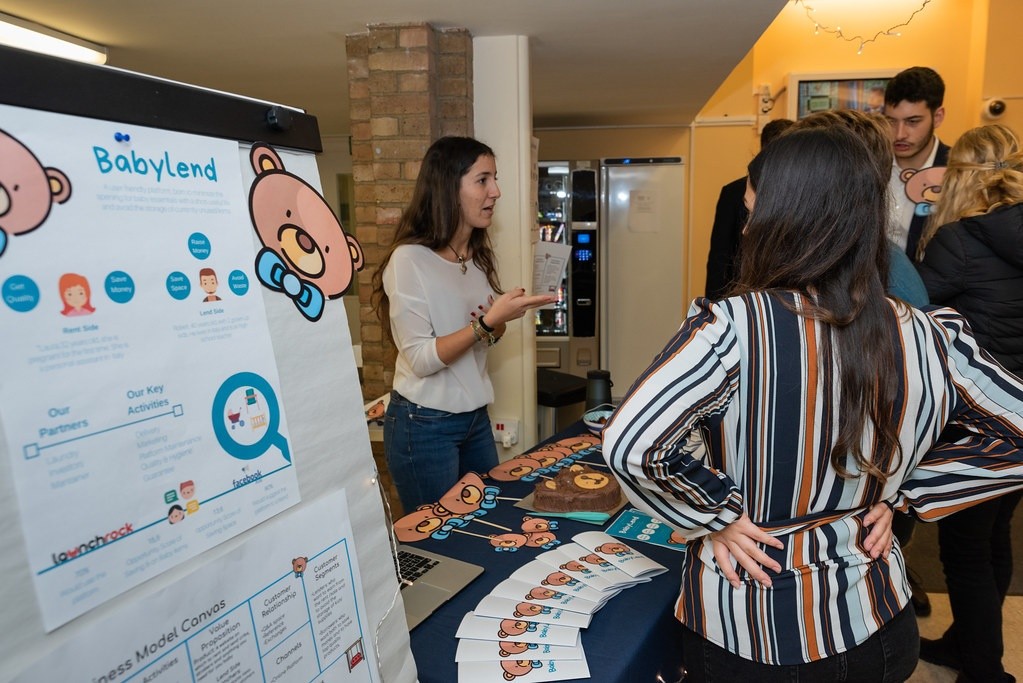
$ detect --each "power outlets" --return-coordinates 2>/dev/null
[492,418,519,444]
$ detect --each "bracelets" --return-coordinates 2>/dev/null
[468,315,498,349]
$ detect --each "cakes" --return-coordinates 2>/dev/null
[535,465,622,513]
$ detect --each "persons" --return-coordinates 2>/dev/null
[602,62,1023,683]
[370,133,559,513]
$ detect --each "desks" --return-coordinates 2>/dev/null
[390,419,691,683]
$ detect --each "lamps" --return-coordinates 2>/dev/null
[0,11,110,67]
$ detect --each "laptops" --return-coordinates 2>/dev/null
[384,513,485,633]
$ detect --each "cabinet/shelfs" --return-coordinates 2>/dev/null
[537,367,589,442]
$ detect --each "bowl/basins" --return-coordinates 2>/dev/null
[581,410,617,436]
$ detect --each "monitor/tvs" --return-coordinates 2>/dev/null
[786,68,904,125]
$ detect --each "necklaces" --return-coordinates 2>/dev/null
[445,239,472,275]
